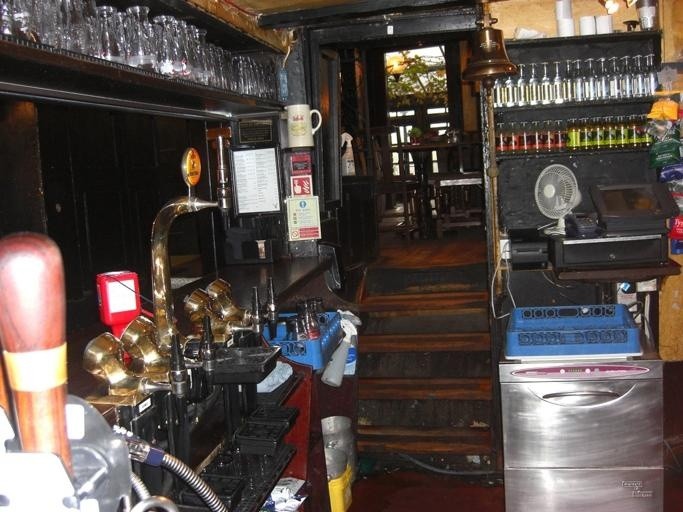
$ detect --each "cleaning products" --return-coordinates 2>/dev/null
[321,309,362,387]
[340,133,357,176]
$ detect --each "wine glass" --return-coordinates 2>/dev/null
[0,0,280,99]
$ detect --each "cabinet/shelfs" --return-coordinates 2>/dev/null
[262,312,359,497]
[486,26,662,165]
[166,338,303,512]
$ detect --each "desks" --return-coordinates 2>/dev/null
[553,260,682,358]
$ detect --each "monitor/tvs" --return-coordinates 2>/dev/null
[589,181,680,232]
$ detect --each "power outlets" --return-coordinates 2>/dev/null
[499,238,512,261]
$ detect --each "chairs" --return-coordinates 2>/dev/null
[341,121,486,242]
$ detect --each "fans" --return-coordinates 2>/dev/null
[529,162,586,237]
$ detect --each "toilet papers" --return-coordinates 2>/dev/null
[555,1,613,37]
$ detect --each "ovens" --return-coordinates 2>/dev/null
[497,336,666,510]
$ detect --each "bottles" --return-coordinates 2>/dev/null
[496,114,657,157]
[494,51,658,106]
[288,296,326,341]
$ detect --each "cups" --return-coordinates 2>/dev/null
[283,103,322,148]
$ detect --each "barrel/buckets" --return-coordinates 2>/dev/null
[636,0,659,31]
[323,448,353,512]
[322,415,355,479]
